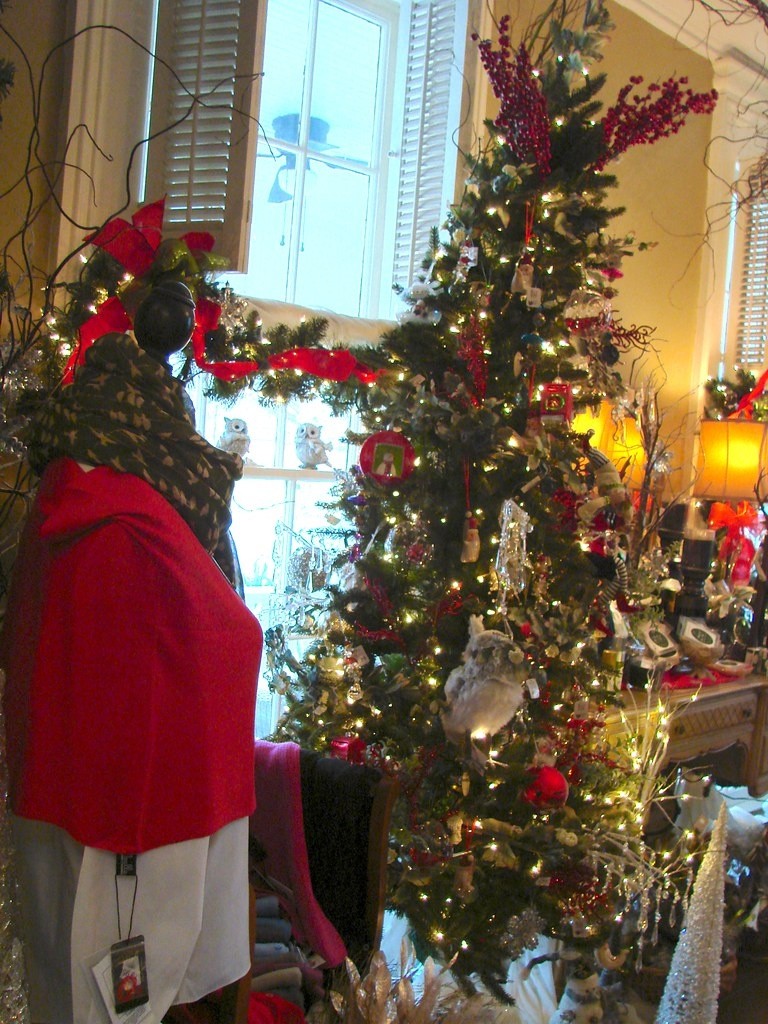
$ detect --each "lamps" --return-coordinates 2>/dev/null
[693,419,768,578]
[571,399,655,529]
[268,155,310,252]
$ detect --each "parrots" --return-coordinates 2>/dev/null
[440,629,523,776]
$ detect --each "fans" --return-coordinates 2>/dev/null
[255,113,368,176]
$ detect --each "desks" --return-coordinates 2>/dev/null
[604,674,768,798]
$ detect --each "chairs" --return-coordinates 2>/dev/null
[249,740,399,977]
